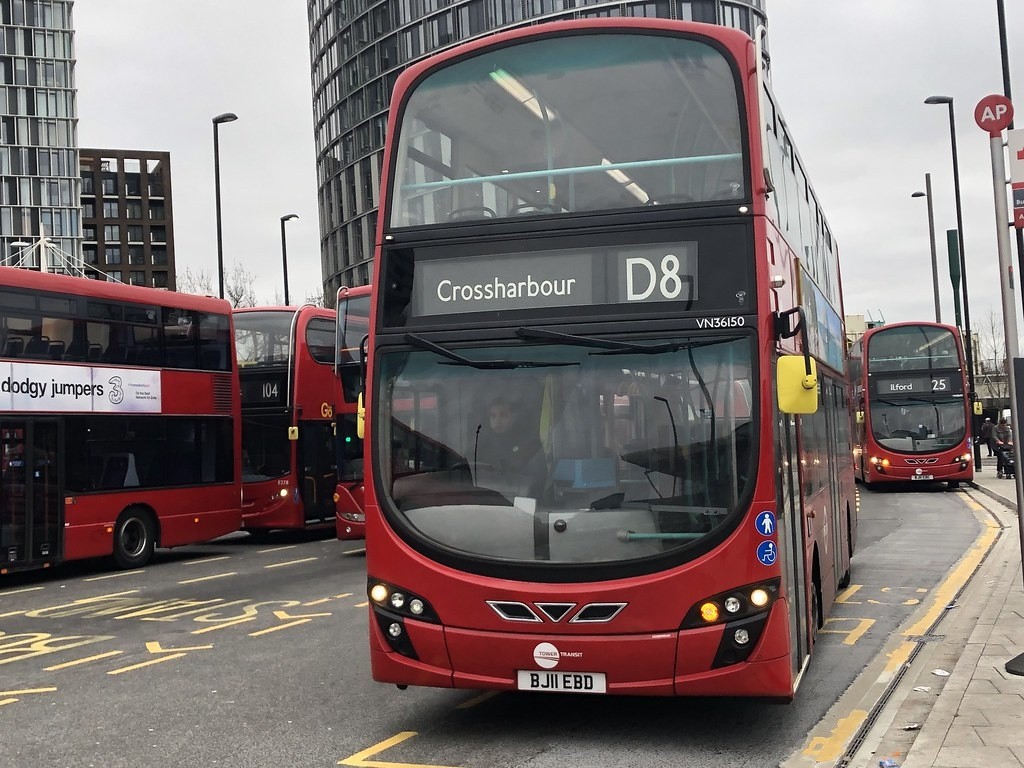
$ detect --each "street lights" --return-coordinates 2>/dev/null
[281,214,301,305]
[212,112,238,300]
[924,96,981,472]
[911,173,941,324]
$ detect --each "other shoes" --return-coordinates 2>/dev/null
[988,455,992,457]
[998,472,1001,478]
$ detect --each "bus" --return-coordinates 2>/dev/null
[235,303,368,538]
[1,264,244,577]
[337,16,858,699]
[330,285,631,540]
[847,321,983,491]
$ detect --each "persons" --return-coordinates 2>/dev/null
[980,418,997,457]
[465,397,548,502]
[991,417,1014,479]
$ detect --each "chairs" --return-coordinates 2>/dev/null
[0,335,222,371]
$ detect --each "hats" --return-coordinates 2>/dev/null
[999,417,1007,422]
[986,417,990,420]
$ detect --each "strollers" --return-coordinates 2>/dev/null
[993,442,1016,479]
